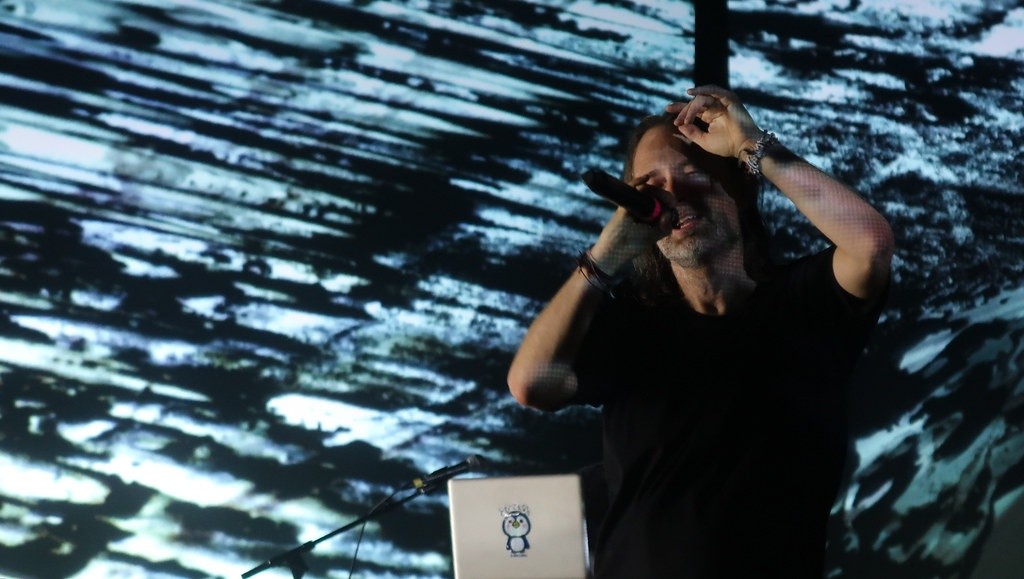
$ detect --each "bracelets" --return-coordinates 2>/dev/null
[737,129,780,175]
[577,244,617,292]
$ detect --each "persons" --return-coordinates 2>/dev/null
[507,84,894,579]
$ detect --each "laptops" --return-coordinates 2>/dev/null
[447,474,594,579]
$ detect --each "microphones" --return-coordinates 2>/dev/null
[581,170,679,229]
[403,454,484,490]
